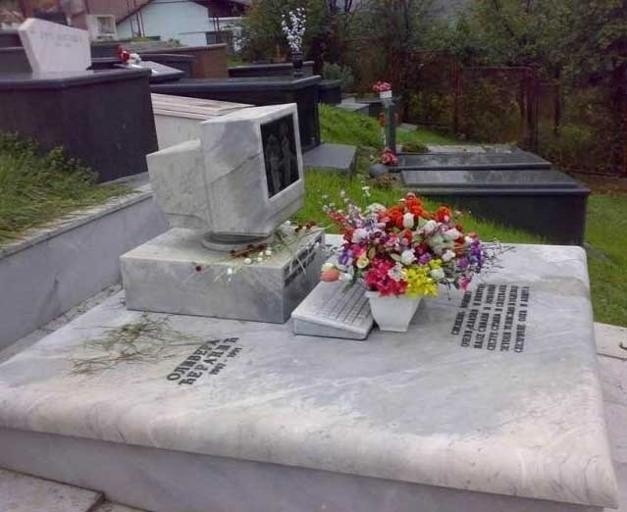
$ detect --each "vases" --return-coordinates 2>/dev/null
[364,291,420,332]
[380,90,392,99]
[293,53,304,77]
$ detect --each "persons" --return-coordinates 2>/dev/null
[265,134,296,193]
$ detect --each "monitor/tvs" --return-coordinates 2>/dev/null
[146,101,306,252]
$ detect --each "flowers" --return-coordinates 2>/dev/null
[317,187,513,299]
[381,148,397,164]
[373,81,392,91]
[281,6,308,52]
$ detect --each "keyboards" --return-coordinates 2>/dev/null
[290,274,374,340]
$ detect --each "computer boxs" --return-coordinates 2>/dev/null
[118,228,325,323]
